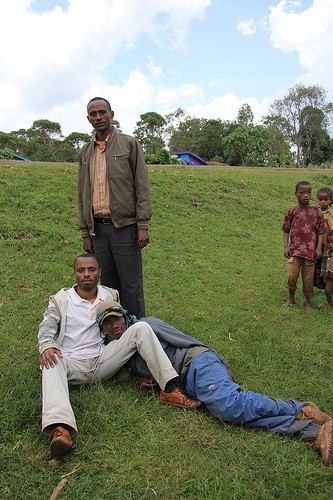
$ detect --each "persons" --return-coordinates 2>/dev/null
[78,97,152,319]
[96,301,333,466]
[324,230,333,308]
[282,181,324,309]
[313,187,333,290]
[37,254,201,458]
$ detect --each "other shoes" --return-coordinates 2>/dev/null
[135,377,158,390]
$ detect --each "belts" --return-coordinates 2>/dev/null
[94,217,114,226]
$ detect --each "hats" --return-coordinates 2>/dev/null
[96,301,124,326]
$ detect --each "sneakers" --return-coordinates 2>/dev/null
[300,401,332,426]
[159,386,201,409]
[307,421,333,466]
[48,426,76,457]
